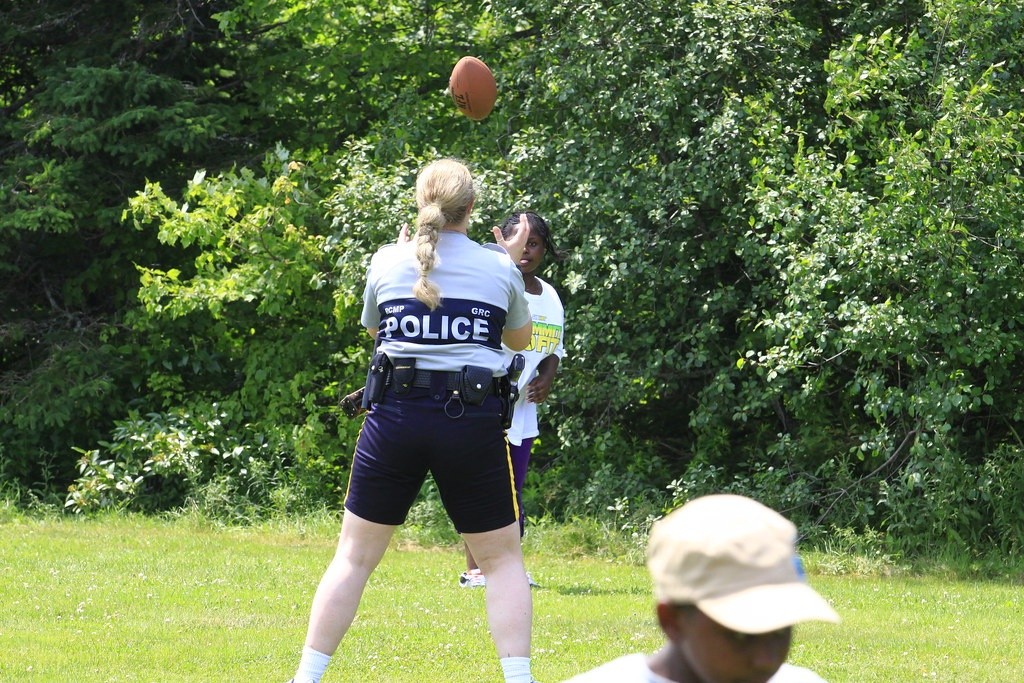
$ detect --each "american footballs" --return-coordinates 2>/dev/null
[448,56,499,122]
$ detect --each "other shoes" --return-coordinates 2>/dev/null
[524,572,541,589]
[458,570,486,589]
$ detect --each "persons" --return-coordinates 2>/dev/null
[560,490,833,683]
[457,207,565,589]
[291,157,535,683]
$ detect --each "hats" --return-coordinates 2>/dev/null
[645,493,843,634]
